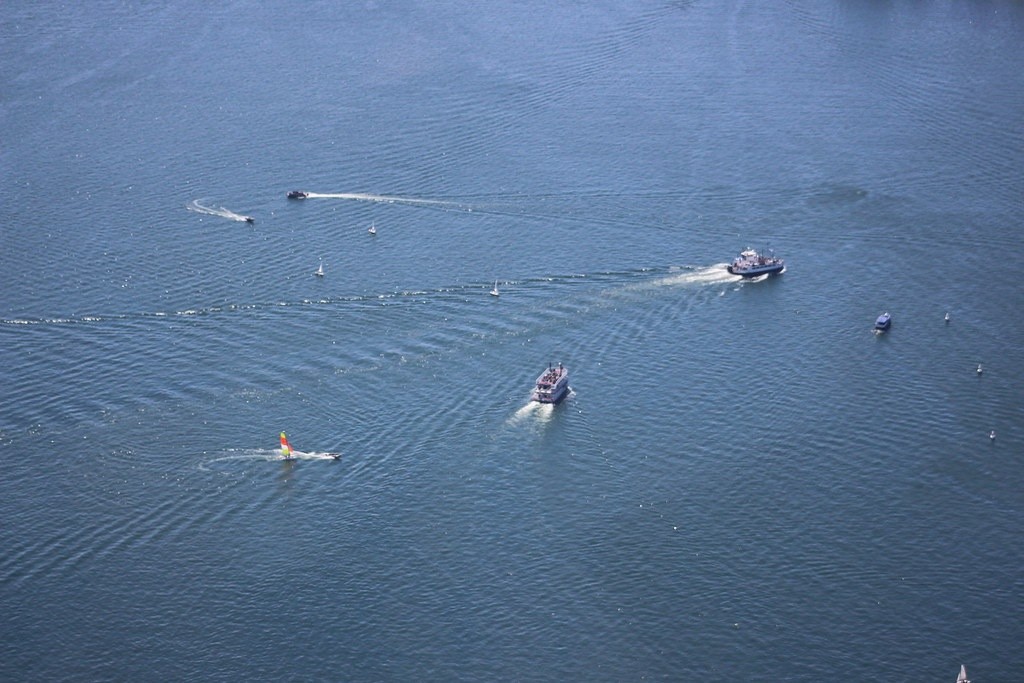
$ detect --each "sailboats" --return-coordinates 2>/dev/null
[368,219,378,234]
[314,263,325,276]
[278,430,300,461]
[489,279,500,296]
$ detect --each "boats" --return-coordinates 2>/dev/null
[245,217,256,223]
[875,312,892,331]
[326,452,342,459]
[533,362,569,402]
[286,189,306,200]
[726,245,785,279]
